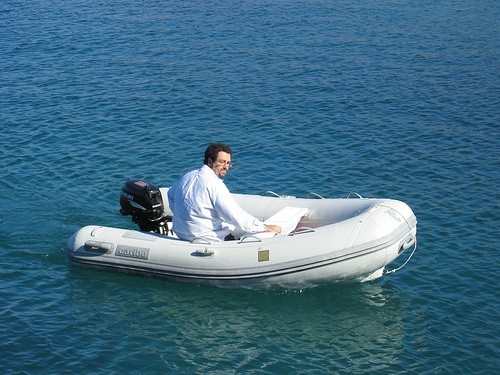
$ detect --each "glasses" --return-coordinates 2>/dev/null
[216,160,231,167]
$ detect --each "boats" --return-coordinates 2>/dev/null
[63,180,421,292]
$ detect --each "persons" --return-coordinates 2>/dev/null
[166,139,283,242]
